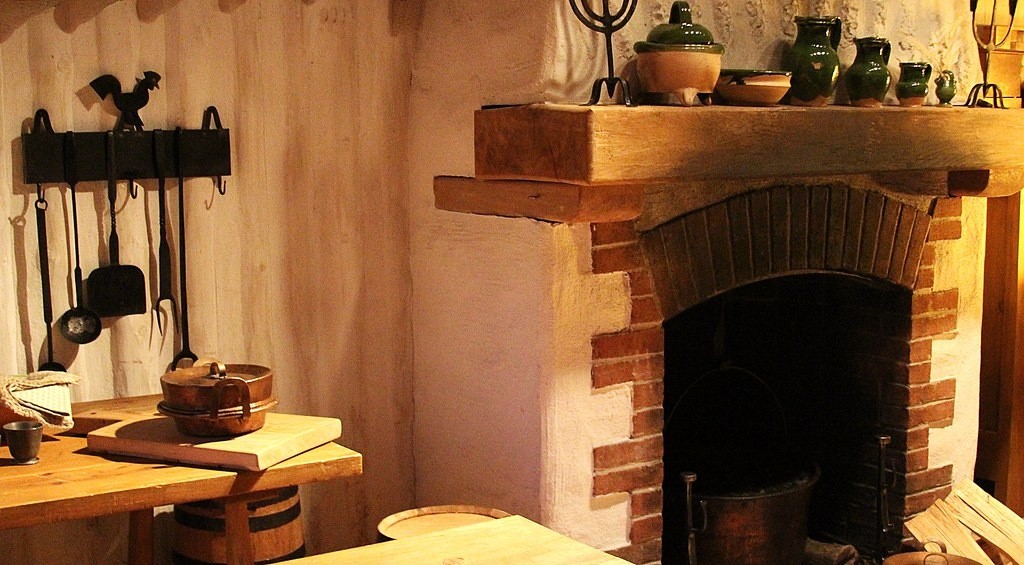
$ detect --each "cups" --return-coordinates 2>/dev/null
[787,15,843,107]
[844,36,893,107]
[894,61,932,107]
[2,420,44,465]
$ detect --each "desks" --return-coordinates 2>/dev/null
[0,393,365,565]
[272,513,639,565]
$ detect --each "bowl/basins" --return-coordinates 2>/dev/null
[716,68,793,106]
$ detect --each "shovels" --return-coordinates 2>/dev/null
[87,178,147,319]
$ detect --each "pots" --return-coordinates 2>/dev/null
[156,356,280,437]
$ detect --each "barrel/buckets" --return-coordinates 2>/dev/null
[683,448,822,565]
[375,503,511,541]
[173,485,306,565]
